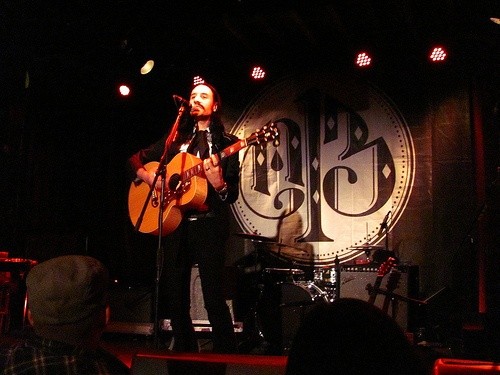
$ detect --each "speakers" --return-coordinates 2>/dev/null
[336,263,419,334]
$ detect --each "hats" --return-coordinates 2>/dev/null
[26,255,111,324]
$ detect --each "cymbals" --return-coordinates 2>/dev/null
[236,233,279,242]
[350,245,384,250]
[256,243,308,256]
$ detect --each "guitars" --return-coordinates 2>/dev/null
[127,120,281,237]
[368,257,397,306]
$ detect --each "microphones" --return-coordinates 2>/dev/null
[172,95,192,110]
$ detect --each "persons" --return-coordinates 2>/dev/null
[285,298,417,375]
[0,255,133,375]
[128,82,240,354]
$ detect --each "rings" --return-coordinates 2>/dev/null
[206,168,210,171]
[214,162,218,166]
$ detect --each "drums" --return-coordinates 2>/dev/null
[312,266,337,286]
[251,281,331,351]
[261,267,307,284]
[317,286,337,303]
